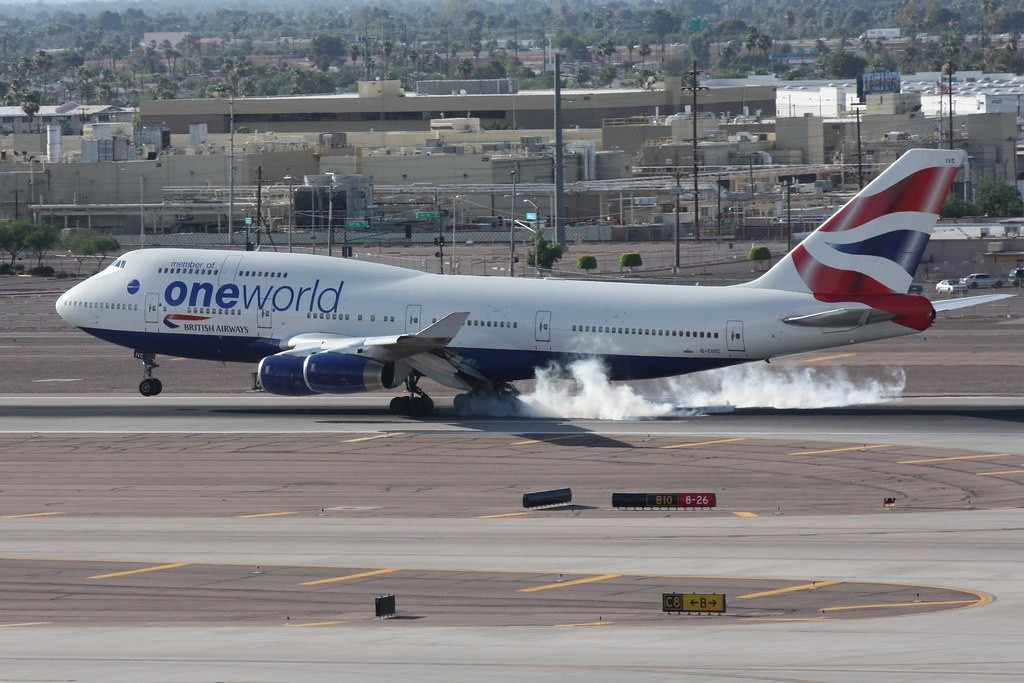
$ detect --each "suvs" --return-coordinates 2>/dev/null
[1008,268,1024,289]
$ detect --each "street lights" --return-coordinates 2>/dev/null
[510,170,515,276]
[250,165,262,247]
[522,199,540,278]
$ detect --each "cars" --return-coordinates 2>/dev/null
[960,272,1005,290]
[907,284,924,295]
[936,279,968,295]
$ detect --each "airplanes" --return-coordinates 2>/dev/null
[56,148,967,423]
